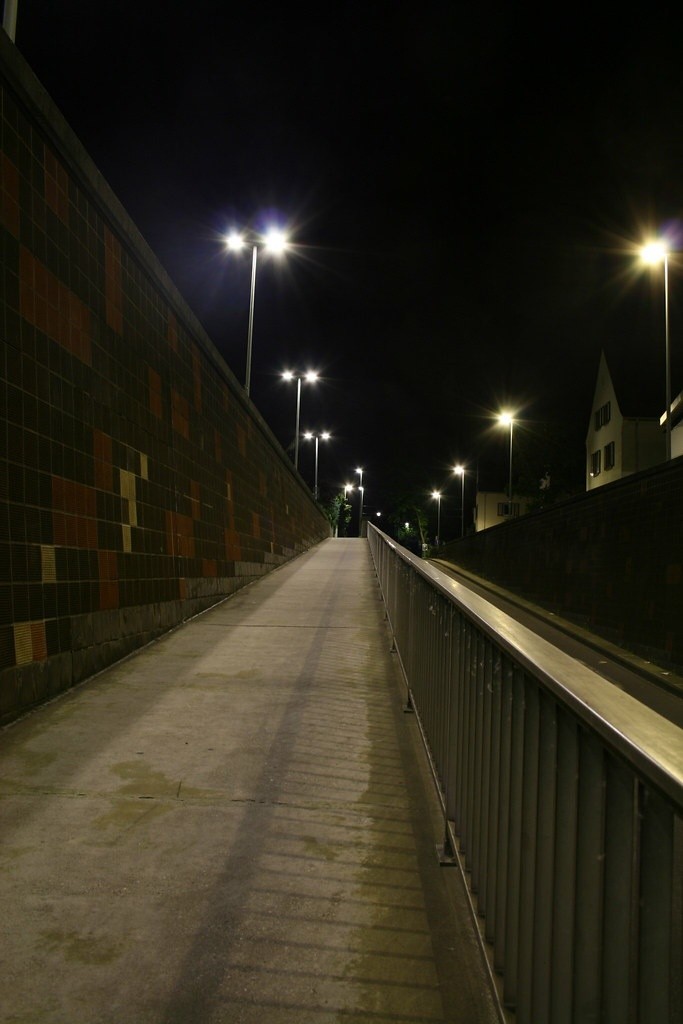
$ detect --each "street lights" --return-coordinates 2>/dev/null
[639,238,672,462]
[304,431,330,500]
[454,465,464,538]
[356,468,363,487]
[433,491,440,547]
[226,229,288,397]
[358,486,364,521]
[345,485,352,498]
[497,411,513,521]
[281,369,317,468]
